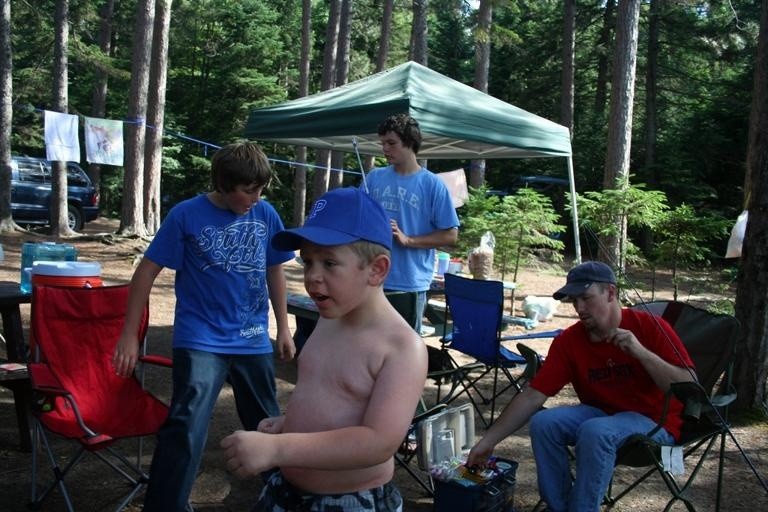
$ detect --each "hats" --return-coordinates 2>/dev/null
[271,185,393,253]
[554,262,617,300]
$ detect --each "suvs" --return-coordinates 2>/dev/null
[10,156,101,232]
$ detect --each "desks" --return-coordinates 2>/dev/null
[0,279,46,455]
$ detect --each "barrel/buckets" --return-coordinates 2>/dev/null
[27,260,104,364]
[438,251,450,274]
[20,240,77,293]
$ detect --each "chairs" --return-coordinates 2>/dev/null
[24,283,173,512]
[394,272,768,512]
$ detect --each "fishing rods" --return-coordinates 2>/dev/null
[582,219,767,493]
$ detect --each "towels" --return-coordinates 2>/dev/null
[436,168,469,210]
[42,108,81,165]
[83,116,125,168]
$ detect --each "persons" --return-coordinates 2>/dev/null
[220,188,428,512]
[467,261,698,512]
[111,142,297,512]
[359,115,460,455]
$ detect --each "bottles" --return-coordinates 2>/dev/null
[438,251,463,274]
